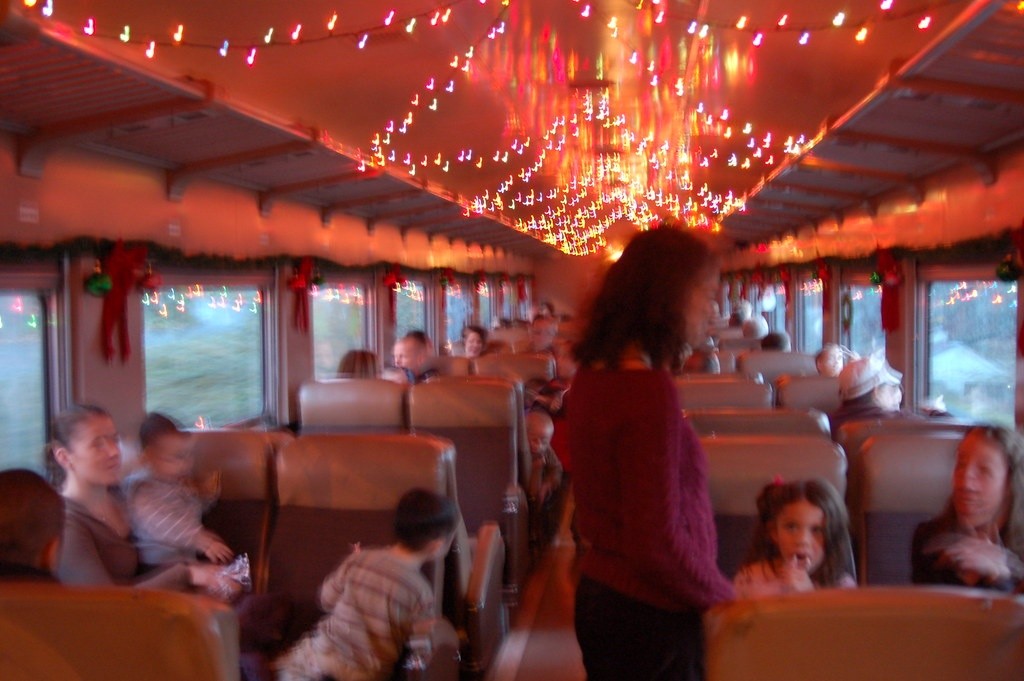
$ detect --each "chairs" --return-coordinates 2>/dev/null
[671,315,1023,681]
[0,313,584,681]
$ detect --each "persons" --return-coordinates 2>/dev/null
[491,300,566,414]
[814,342,844,378]
[43,400,272,681]
[336,329,443,391]
[910,425,1024,595]
[272,486,459,681]
[828,348,952,444]
[1,468,66,584]
[460,325,487,359]
[706,296,791,353]
[566,226,738,681]
[523,411,563,501]
[731,476,859,594]
